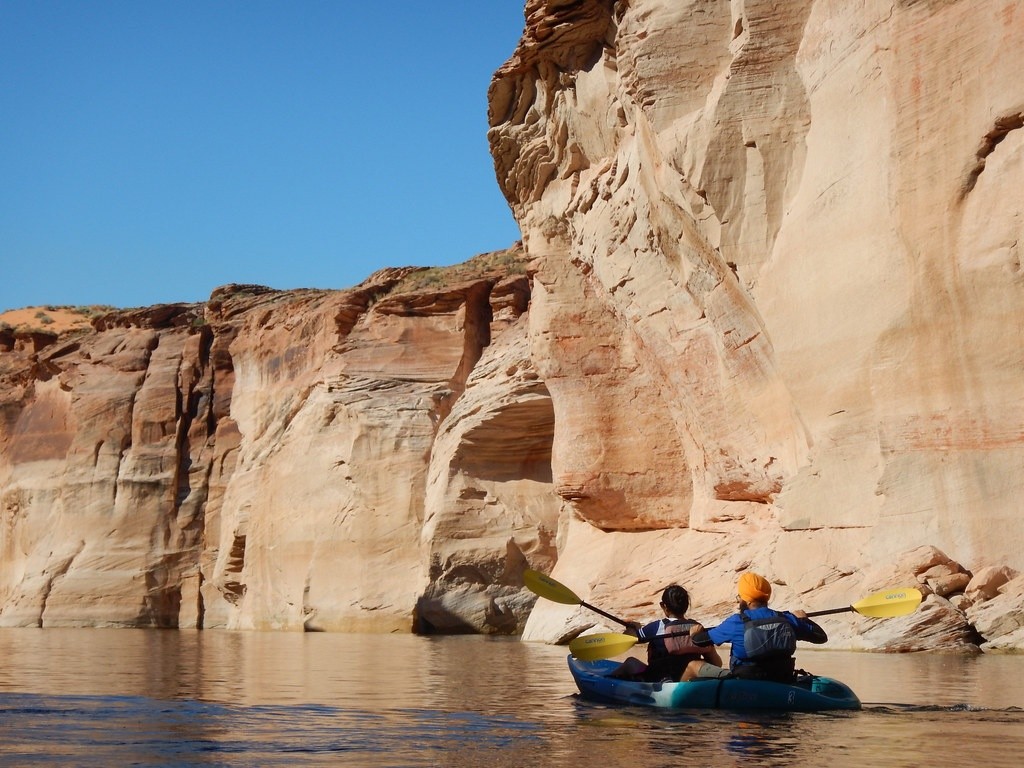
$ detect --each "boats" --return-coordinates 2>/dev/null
[566,653,862,715]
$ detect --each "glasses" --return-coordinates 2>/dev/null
[660,602,665,608]
[736,594,741,602]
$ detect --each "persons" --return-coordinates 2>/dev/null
[679,571,828,683]
[611,584,723,682]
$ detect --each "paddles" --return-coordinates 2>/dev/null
[567,587,924,663]
[522,568,636,630]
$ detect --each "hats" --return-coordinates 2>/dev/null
[737,571,771,604]
